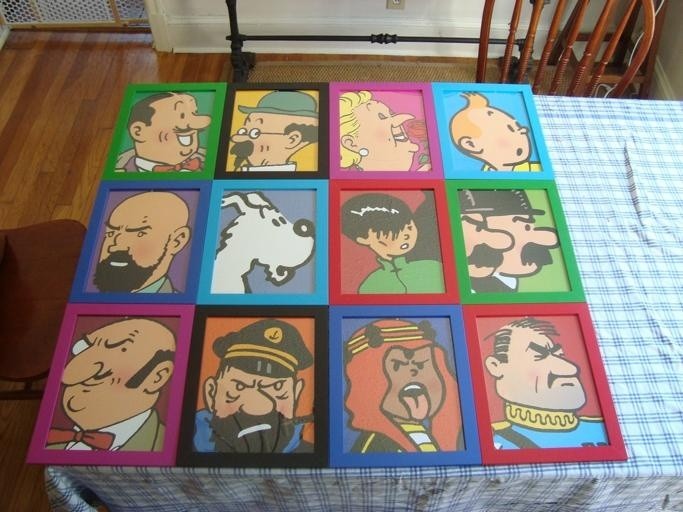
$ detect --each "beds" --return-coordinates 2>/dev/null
[43,95,683,512]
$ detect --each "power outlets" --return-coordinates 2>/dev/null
[386,0,404,11]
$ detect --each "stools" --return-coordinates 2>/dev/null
[546,1,670,99]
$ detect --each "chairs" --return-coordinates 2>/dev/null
[0,219,86,398]
[476,1,656,101]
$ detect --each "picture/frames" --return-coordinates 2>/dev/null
[197,179,329,307]
[432,81,556,180]
[463,303,627,466]
[176,306,329,469]
[446,180,586,304]
[328,180,460,304]
[101,82,227,178]
[214,83,329,180]
[25,304,196,467]
[330,81,445,179]
[68,179,210,304]
[328,305,482,468]
